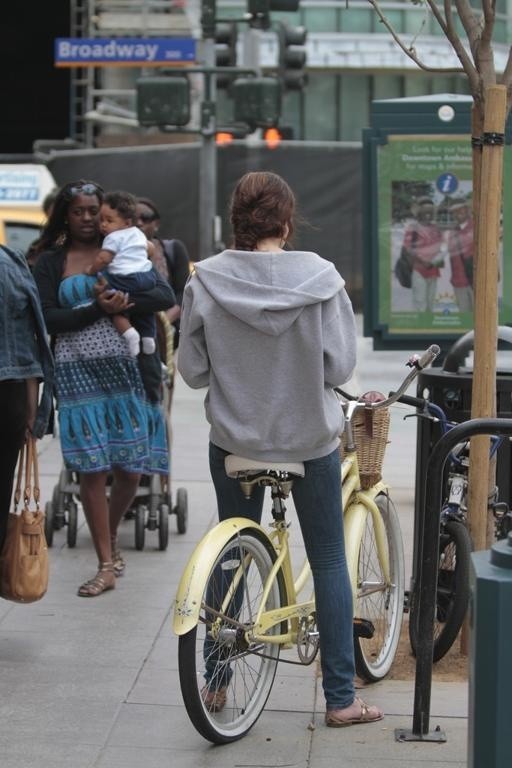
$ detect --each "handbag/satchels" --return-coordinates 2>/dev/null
[0,508,50,603]
[394,247,414,288]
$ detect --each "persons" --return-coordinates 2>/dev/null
[0,174,194,599]
[393,198,444,327]
[446,197,474,314]
[178,171,384,729]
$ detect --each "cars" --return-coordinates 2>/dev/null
[1,162,198,278]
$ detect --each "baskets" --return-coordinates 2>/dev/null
[336,403,391,489]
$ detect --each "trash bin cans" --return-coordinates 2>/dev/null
[413,324,512,621]
[466,532,512,766]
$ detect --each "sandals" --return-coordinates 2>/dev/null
[77,537,126,596]
[326,695,385,728]
[200,684,227,712]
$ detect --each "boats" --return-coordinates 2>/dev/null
[246,0,300,13]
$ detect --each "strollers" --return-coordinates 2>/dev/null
[46,309,190,550]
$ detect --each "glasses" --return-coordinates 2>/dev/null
[133,211,156,225]
[64,184,97,202]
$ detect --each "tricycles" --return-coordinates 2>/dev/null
[388,325,511,664]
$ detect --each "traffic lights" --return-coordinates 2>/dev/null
[214,22,235,85]
[286,26,309,89]
[260,124,295,152]
[217,123,249,145]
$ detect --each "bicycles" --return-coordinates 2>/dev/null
[174,337,441,747]
